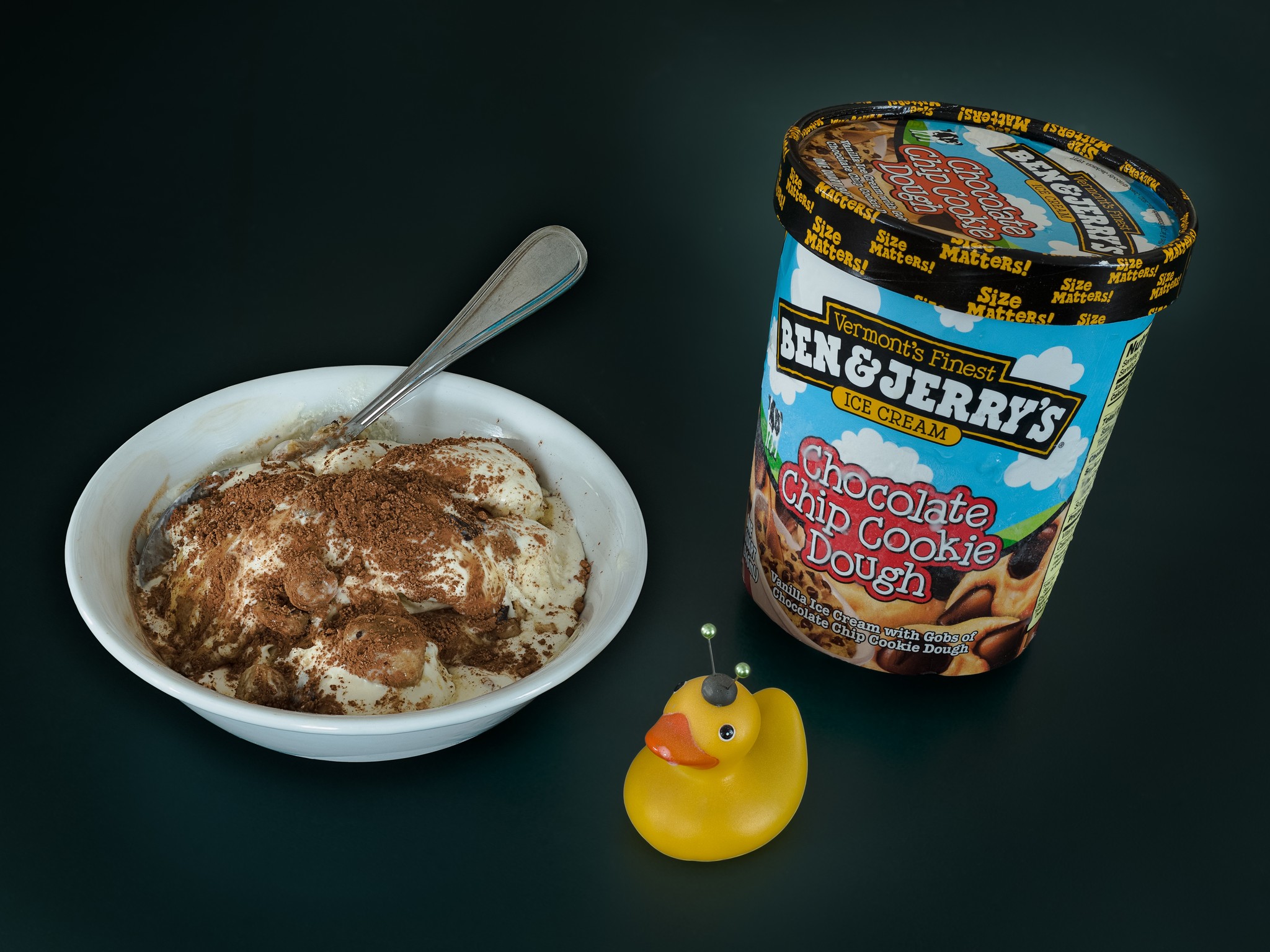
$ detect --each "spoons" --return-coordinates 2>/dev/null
[136,225,588,587]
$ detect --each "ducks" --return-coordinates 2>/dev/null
[623,670,809,861]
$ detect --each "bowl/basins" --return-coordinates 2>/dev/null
[63,366,649,763]
